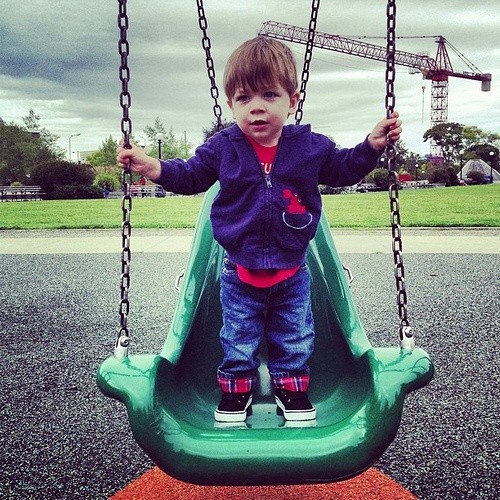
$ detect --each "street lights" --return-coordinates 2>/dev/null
[69,134,80,163]
[156,132,163,159]
[459,149,464,180]
[489,152,494,183]
[415,164,419,181]
[381,157,385,169]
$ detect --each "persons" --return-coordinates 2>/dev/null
[104,177,151,197]
[116,36,402,423]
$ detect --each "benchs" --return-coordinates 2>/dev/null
[129,184,164,198]
[0,184,47,202]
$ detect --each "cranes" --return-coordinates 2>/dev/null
[257,20,492,181]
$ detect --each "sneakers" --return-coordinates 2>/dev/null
[274,389,316,420]
[214,392,253,422]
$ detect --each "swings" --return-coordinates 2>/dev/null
[92,1,440,489]
